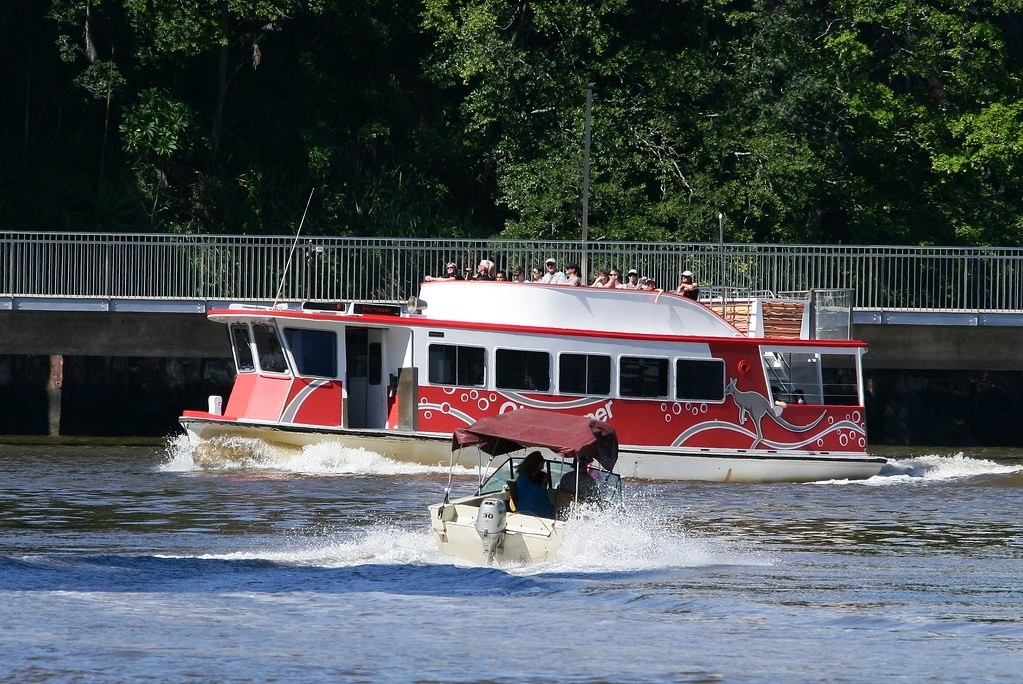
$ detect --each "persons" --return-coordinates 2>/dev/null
[559,458,596,497]
[565,262,582,284]
[770,386,786,406]
[591,269,610,287]
[465,260,496,281]
[512,266,530,282]
[622,269,645,289]
[259,338,287,371]
[793,389,805,404]
[425,263,465,281]
[539,258,567,284]
[864,378,874,400]
[533,265,544,282]
[604,269,624,287]
[676,271,697,298]
[496,271,507,281]
[637,278,664,303]
[516,451,547,512]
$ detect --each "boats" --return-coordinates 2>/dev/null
[175,186,892,487]
[427,408,643,566]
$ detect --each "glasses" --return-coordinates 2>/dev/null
[629,273,637,277]
[546,262,555,266]
[446,266,454,269]
[513,272,523,277]
[608,273,617,276]
[682,275,689,278]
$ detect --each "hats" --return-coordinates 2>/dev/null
[564,262,578,271]
[645,278,655,284]
[681,271,693,279]
[545,258,556,264]
[627,269,637,274]
[570,457,589,469]
